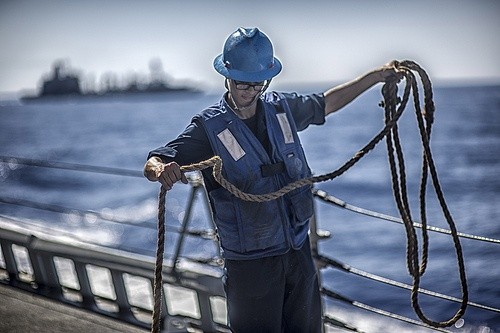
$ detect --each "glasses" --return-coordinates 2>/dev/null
[234,81,268,92]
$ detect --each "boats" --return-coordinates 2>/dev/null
[19,65,204,106]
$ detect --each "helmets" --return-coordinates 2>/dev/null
[214,27,282,82]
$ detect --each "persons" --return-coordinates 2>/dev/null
[143,26,404,333]
